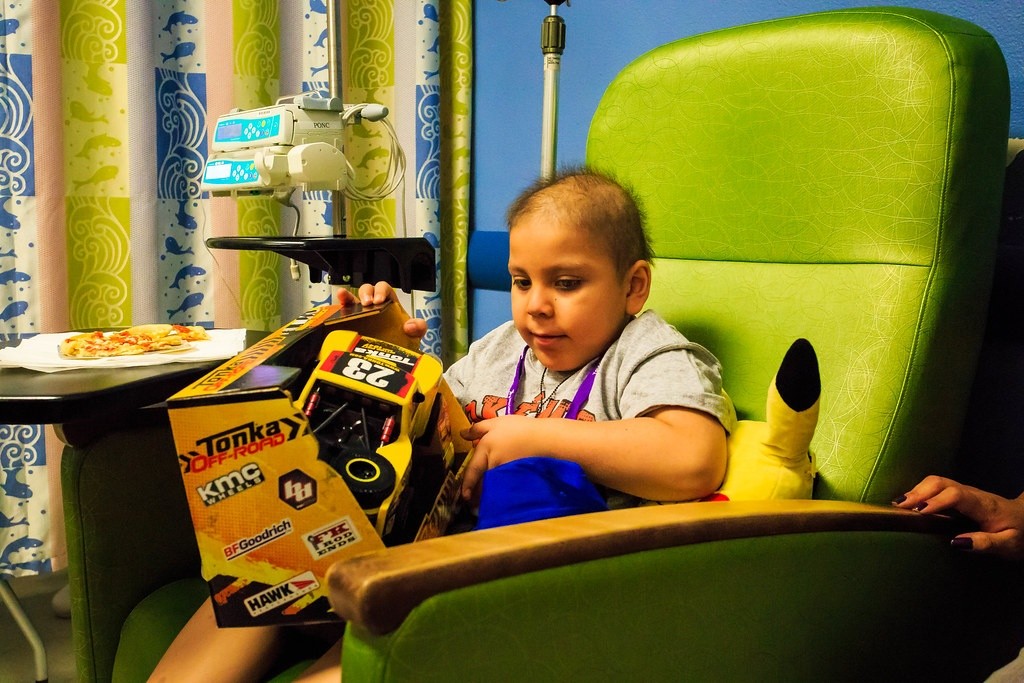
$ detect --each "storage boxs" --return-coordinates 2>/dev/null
[167,298,477,628]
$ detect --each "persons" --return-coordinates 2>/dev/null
[891,476,1024,683]
[147,170,736,683]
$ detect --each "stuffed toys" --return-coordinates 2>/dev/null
[605,337,822,511]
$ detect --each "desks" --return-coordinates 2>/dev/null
[0,328,273,683]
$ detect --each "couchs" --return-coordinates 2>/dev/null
[60,5,1024,683]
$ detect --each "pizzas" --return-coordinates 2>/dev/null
[108,324,185,351]
[171,325,211,339]
[62,329,144,358]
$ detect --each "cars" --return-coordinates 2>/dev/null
[294,329,446,542]
